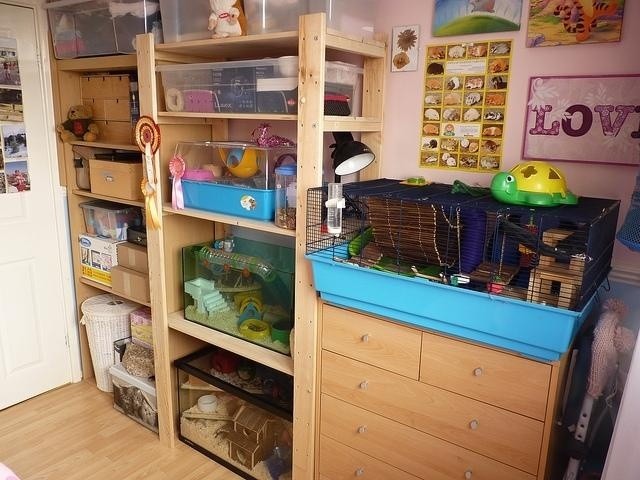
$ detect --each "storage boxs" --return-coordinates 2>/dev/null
[243,0,379,40]
[159,0,216,44]
[41,0,164,59]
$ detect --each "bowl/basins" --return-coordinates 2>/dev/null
[279,56,300,77]
[271,319,294,344]
[239,318,270,339]
[198,393,219,414]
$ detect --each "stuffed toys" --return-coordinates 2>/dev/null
[207,0,242,39]
[57,105,98,141]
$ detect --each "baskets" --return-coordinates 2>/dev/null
[81,294,137,392]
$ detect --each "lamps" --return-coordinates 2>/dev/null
[328,131,376,182]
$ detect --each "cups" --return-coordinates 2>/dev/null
[223,240,234,253]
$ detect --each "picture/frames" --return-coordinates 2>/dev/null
[522,75,639,166]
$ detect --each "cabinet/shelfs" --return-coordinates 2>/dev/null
[148,10,385,479]
[314,296,579,480]
[47,59,146,453]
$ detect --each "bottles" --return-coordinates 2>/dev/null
[328,182,343,235]
[272,164,296,230]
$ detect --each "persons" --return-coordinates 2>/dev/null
[3,58,12,80]
[12,170,27,191]
